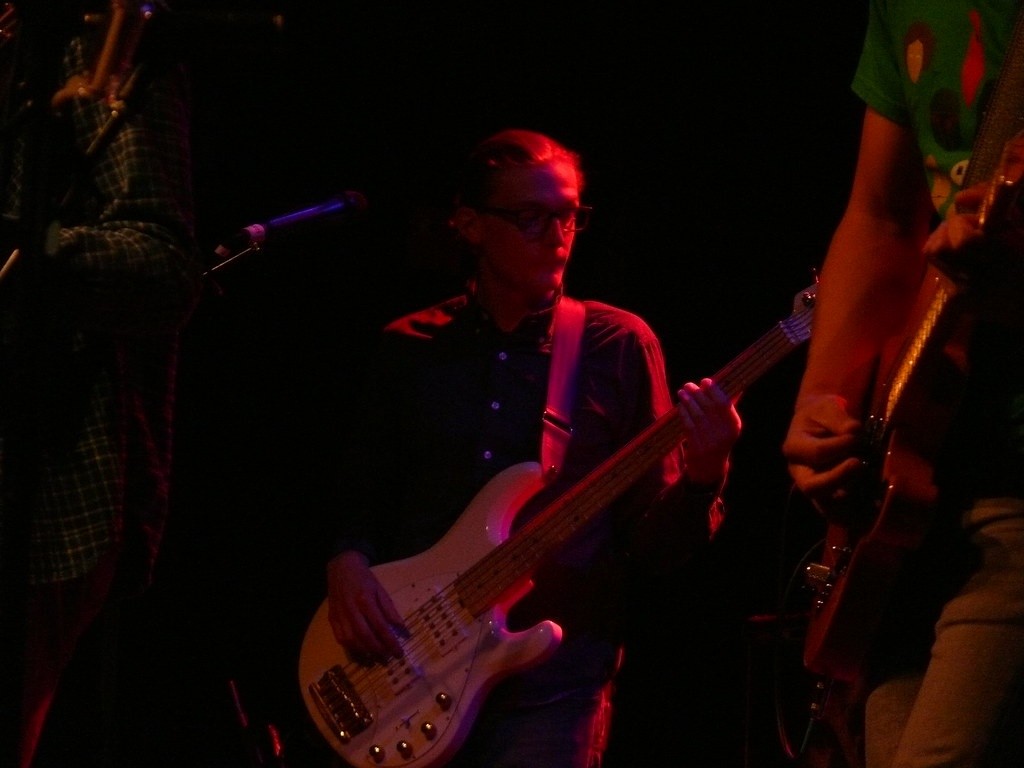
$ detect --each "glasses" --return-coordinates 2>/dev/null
[477,205,593,239]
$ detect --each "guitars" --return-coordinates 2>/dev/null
[783,0,1024,694]
[294,264,830,768]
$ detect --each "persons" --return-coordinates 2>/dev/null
[328,126,744,768]
[0,0,201,768]
[782,0,1023,768]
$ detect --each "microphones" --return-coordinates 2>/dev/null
[90,0,156,99]
[244,191,368,240]
